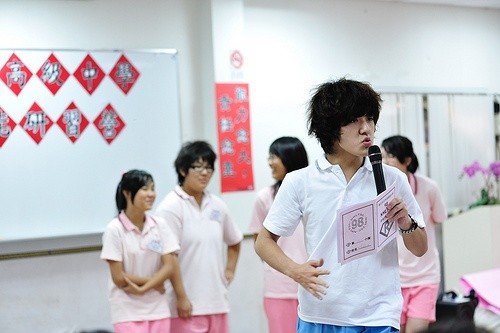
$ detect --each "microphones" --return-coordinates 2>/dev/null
[368,145,386,195]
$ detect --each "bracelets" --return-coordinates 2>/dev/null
[400,214,418,234]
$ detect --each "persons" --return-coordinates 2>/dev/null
[381,135,448,333]
[155,141,242,333]
[101,169,181,333]
[254,76,428,333]
[254,136,309,333]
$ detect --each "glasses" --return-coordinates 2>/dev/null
[187,164,214,173]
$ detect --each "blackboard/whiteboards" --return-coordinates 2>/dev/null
[0,49,183,255]
